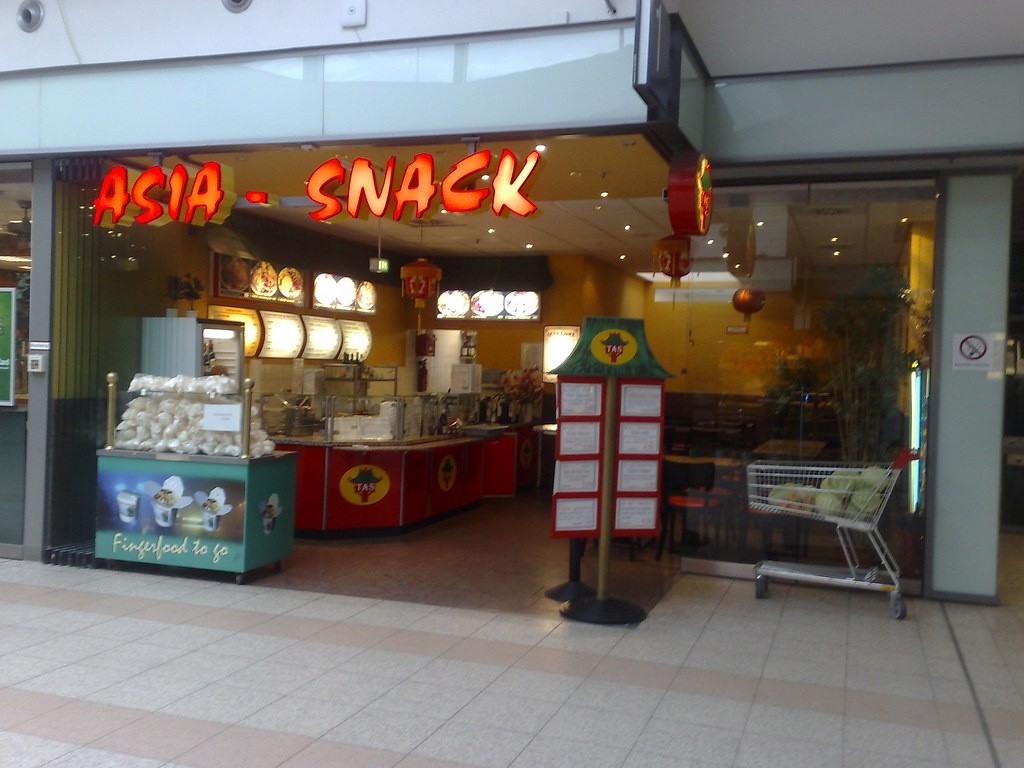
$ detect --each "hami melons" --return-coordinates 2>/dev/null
[766,466,889,522]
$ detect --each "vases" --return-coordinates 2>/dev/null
[187,310,199,318]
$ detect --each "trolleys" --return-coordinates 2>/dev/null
[746,448,920,620]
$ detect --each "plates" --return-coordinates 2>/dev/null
[277,266,303,298]
[505,291,540,319]
[355,280,377,310]
[470,290,505,316]
[251,262,278,298]
[337,276,356,306]
[313,273,337,305]
[219,258,249,293]
[436,290,470,315]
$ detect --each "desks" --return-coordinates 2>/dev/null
[752,437,828,557]
[663,455,748,553]
[322,363,398,415]
[674,426,742,456]
[533,424,557,488]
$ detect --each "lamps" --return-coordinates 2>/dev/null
[7,199,31,242]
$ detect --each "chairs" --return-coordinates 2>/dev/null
[653,460,724,563]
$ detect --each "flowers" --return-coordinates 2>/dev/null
[184,272,205,310]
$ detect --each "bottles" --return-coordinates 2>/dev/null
[354,353,359,364]
[460,333,468,355]
[359,354,364,364]
[349,354,354,364]
[467,332,476,357]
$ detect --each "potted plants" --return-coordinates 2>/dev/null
[165,274,184,317]
